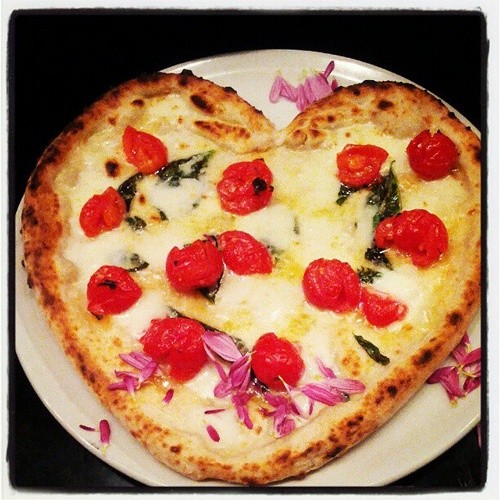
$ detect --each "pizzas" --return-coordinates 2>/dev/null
[20,69,482,485]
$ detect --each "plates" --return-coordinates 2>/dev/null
[16,50,481,487]
